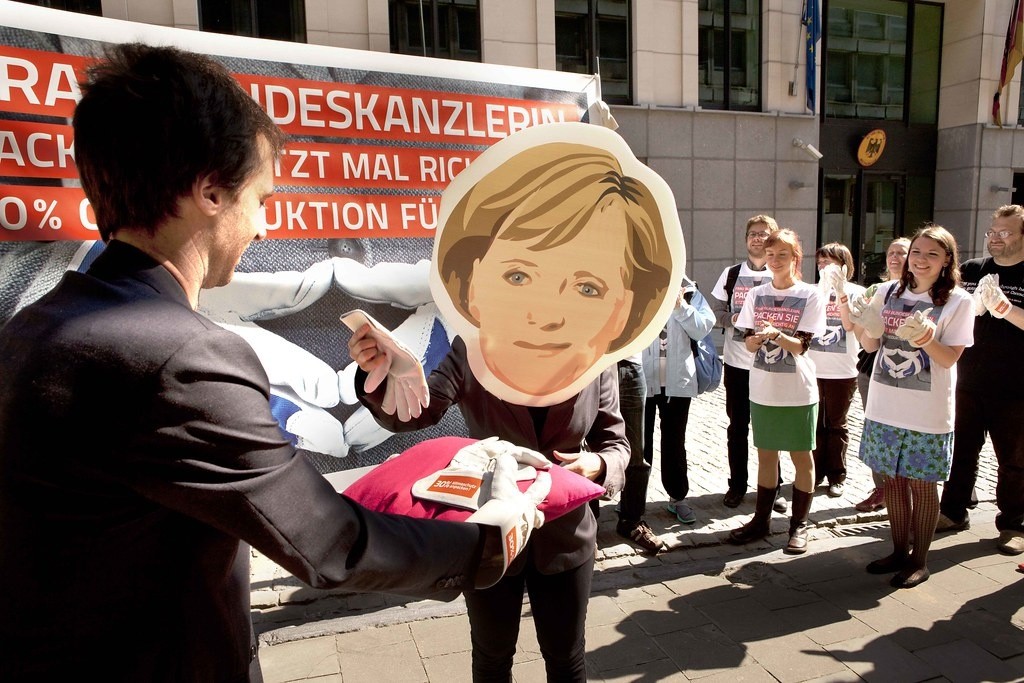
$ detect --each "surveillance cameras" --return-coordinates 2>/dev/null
[805,144,823,160]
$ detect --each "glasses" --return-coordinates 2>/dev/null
[985,230,1024,239]
[746,231,769,239]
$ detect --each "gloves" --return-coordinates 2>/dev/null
[756,322,928,380]
[461,452,554,589]
[980,273,1012,319]
[816,262,837,305]
[72,237,459,458]
[340,308,429,423]
[411,436,552,512]
[970,273,995,317]
[896,308,938,348]
[849,292,886,339]
[829,265,849,306]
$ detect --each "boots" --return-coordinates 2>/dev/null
[729,485,779,542]
[786,485,815,554]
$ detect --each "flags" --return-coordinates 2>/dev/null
[805,0,821,116]
[992,0,1024,130]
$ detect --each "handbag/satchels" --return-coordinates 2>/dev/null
[856,279,901,380]
[684,279,723,395]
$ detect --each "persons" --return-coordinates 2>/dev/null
[811,243,865,496]
[710,213,788,512]
[582,350,663,552]
[728,229,823,553]
[848,225,975,588]
[0,40,551,683]
[614,275,717,523]
[348,323,633,683]
[0,24,591,479]
[934,203,1024,554]
[854,236,910,512]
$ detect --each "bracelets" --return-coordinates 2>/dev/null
[774,332,782,340]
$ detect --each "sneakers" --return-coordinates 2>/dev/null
[855,485,888,512]
[667,496,697,524]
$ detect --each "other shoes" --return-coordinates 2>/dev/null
[772,494,788,512]
[996,529,1024,554]
[723,486,746,509]
[828,480,843,496]
[866,553,911,574]
[612,507,663,555]
[888,564,931,588]
[935,513,970,533]
